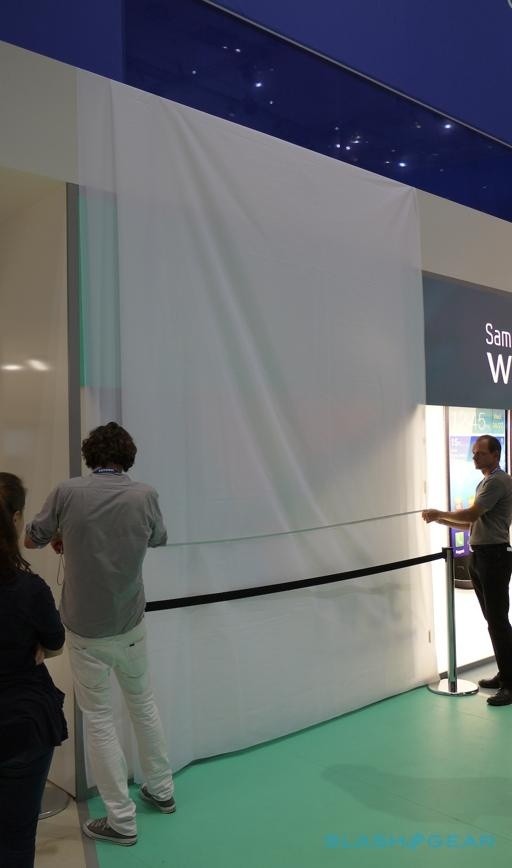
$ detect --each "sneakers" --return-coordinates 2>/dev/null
[141,782,177,815]
[478,672,505,689]
[487,687,511,706]
[82,817,139,848]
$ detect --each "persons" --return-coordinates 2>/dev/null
[23,423,177,849]
[421,435,512,706]
[1,469,70,867]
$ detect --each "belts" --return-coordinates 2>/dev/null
[468,543,510,552]
